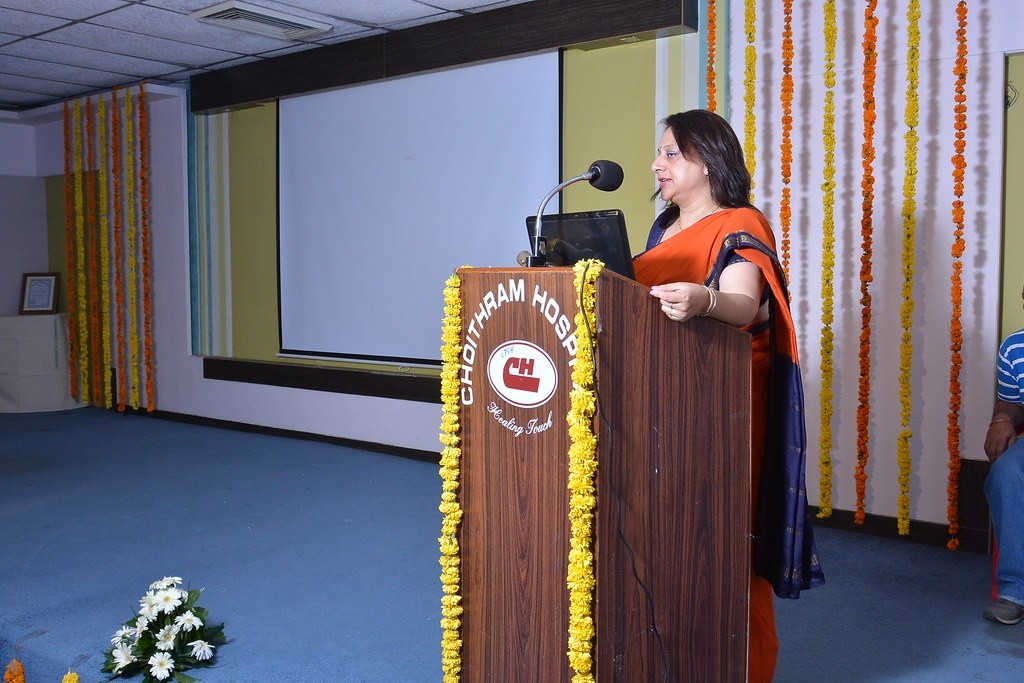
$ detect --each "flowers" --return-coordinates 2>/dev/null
[96,574,232,683]
[3,658,25,683]
[564,258,606,683]
[437,264,474,683]
[704,0,969,551]
[61,672,80,683]
[63,83,158,414]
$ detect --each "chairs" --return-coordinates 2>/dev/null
[990,427,1024,603]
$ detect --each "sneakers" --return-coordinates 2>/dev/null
[983,598,1024,624]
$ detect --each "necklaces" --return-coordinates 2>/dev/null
[679,205,720,230]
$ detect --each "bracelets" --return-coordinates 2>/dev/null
[989,420,1015,427]
[697,286,717,317]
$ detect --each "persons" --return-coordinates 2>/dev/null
[983,284,1024,626]
[631,109,825,683]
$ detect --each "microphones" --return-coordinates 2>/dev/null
[526,160,624,269]
[516,251,532,267]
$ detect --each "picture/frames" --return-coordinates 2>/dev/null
[18,271,60,315]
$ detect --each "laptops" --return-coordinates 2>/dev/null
[526,209,636,282]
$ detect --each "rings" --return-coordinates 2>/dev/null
[671,303,672,309]
[670,309,672,316]
